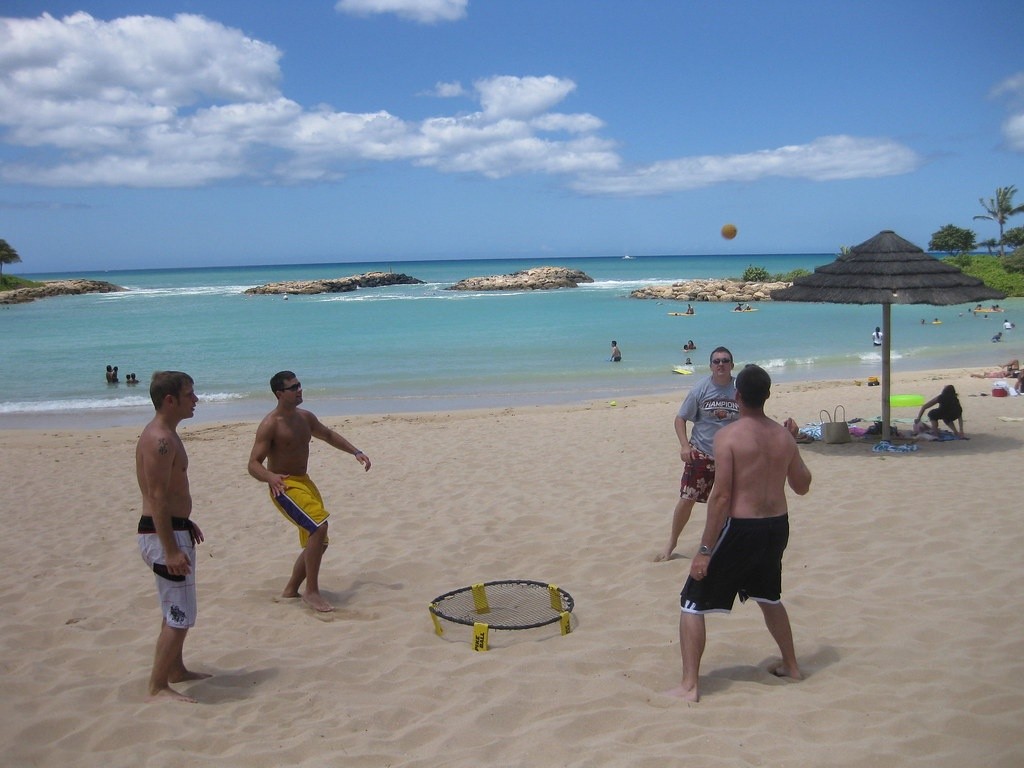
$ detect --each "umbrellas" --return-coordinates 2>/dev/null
[770,230,1013,445]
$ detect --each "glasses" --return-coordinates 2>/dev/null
[712,359,730,363]
[283,383,301,391]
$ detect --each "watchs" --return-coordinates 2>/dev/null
[698,545,712,555]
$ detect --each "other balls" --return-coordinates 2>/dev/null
[721,224,737,240]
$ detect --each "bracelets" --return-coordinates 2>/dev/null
[354,450,362,456]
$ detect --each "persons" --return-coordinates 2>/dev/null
[1002,319,1012,329]
[105,365,119,383]
[658,363,810,704]
[134,370,213,703]
[683,340,696,352]
[609,340,622,362]
[125,373,139,384]
[991,332,1003,343]
[871,326,883,346]
[685,358,692,364]
[745,306,751,310]
[686,304,694,316]
[247,371,371,612]
[959,303,999,318]
[970,359,1024,393]
[913,384,964,440]
[654,346,737,561]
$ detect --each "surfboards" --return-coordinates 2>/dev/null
[736,308,759,313]
[668,313,691,315]
[671,369,693,375]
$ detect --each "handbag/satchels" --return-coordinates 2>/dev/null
[820,405,850,443]
